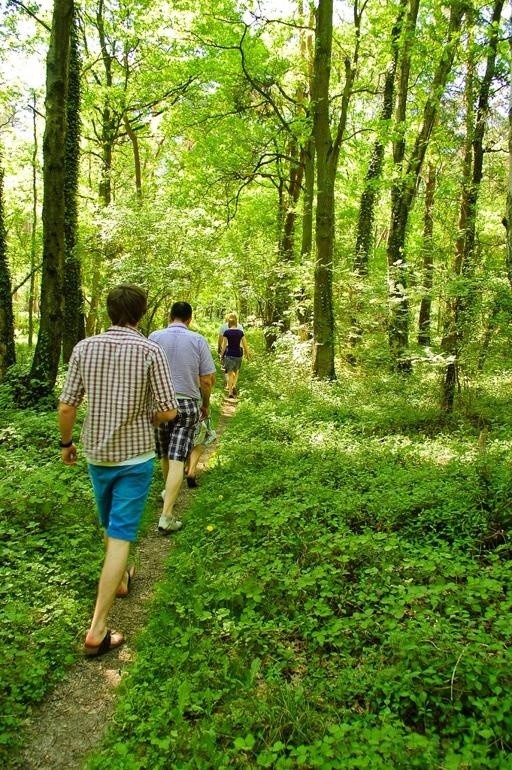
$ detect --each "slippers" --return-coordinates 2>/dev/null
[116,566,135,597]
[84,629,124,657]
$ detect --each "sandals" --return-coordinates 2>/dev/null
[184,468,197,487]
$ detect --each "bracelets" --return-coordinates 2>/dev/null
[59,441,72,447]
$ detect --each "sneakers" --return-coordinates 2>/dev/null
[158,489,182,534]
[224,386,236,398]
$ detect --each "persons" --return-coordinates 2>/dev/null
[58,284,180,658]
[218,313,249,398]
[148,302,216,532]
